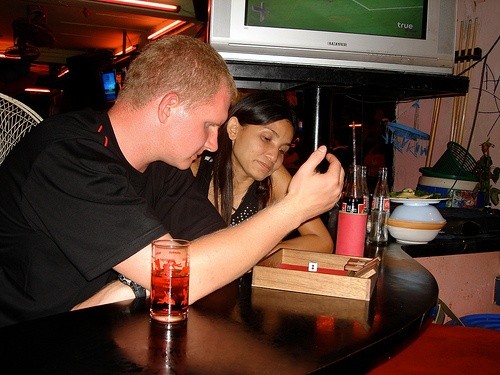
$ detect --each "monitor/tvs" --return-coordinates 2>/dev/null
[211,0,457,76]
[101,69,119,103]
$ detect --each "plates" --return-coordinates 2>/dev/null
[390,198,449,204]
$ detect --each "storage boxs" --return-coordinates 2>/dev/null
[251,247,381,302]
[251,287,368,321]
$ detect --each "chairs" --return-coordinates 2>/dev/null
[0,92,44,166]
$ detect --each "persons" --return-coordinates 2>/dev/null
[191,89,334,256]
[0,34,344,326]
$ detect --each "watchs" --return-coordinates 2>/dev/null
[116,273,147,298]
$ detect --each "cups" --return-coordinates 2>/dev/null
[335,210,367,258]
[148,238,192,329]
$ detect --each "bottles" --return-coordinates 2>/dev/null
[340,165,369,213]
[368,167,390,247]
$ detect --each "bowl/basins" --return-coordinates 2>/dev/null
[387,201,448,244]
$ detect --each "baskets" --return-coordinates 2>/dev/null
[430,141,479,178]
[418,166,479,182]
